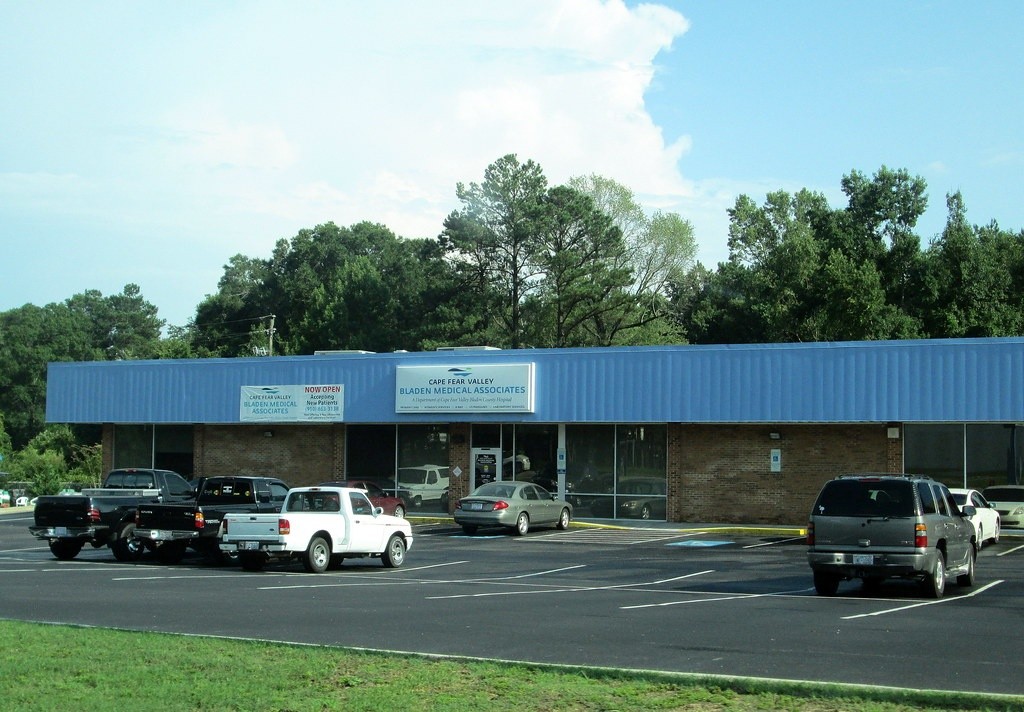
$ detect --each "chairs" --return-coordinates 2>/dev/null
[16,496,28,507]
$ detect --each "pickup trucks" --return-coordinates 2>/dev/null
[218,486,413,574]
[29,468,198,563]
[134,476,308,565]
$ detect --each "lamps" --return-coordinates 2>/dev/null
[769,431,782,440]
[263,429,275,437]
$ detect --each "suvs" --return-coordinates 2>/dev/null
[808,472,978,600]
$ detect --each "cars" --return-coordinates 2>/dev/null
[532,461,618,508]
[980,484,1024,528]
[396,464,451,509]
[302,480,407,518]
[590,476,667,520]
[454,480,574,536]
[947,488,1001,552]
